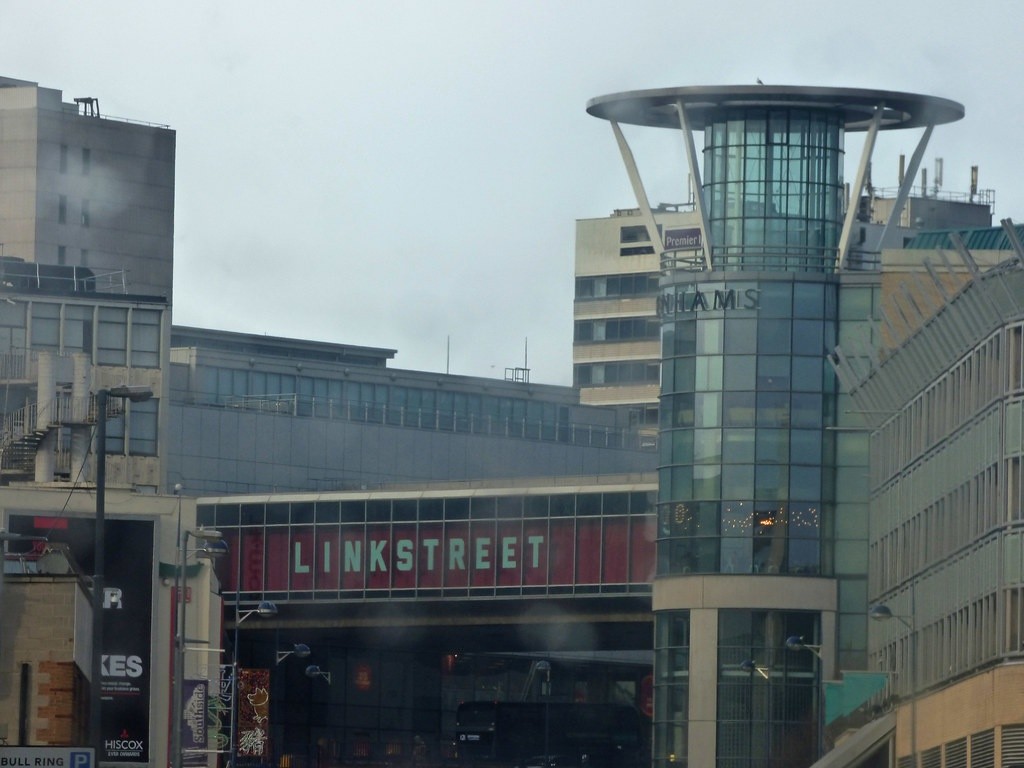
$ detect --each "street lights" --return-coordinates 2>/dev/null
[230,602,278,768]
[738,658,771,768]
[785,635,823,759]
[86,385,154,768]
[171,527,229,768]
[869,605,919,768]
